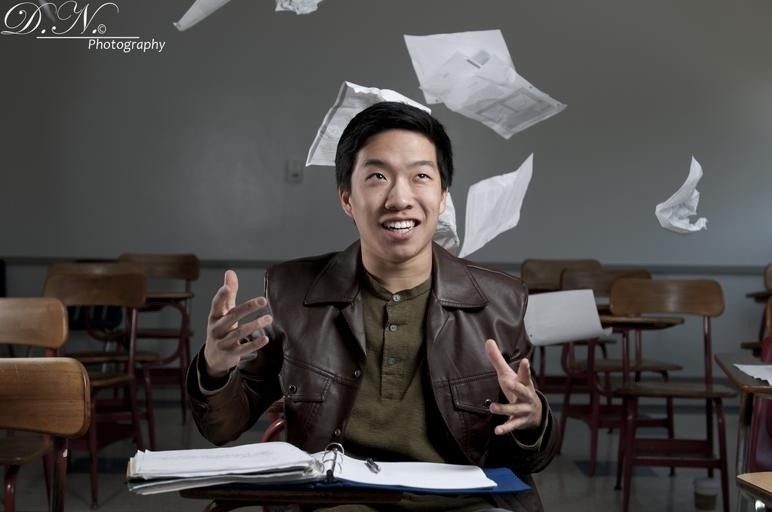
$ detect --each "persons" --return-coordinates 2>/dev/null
[183,100,563,512]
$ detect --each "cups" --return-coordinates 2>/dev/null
[693,478,719,512]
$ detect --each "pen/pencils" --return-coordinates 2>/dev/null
[366,458,381,471]
